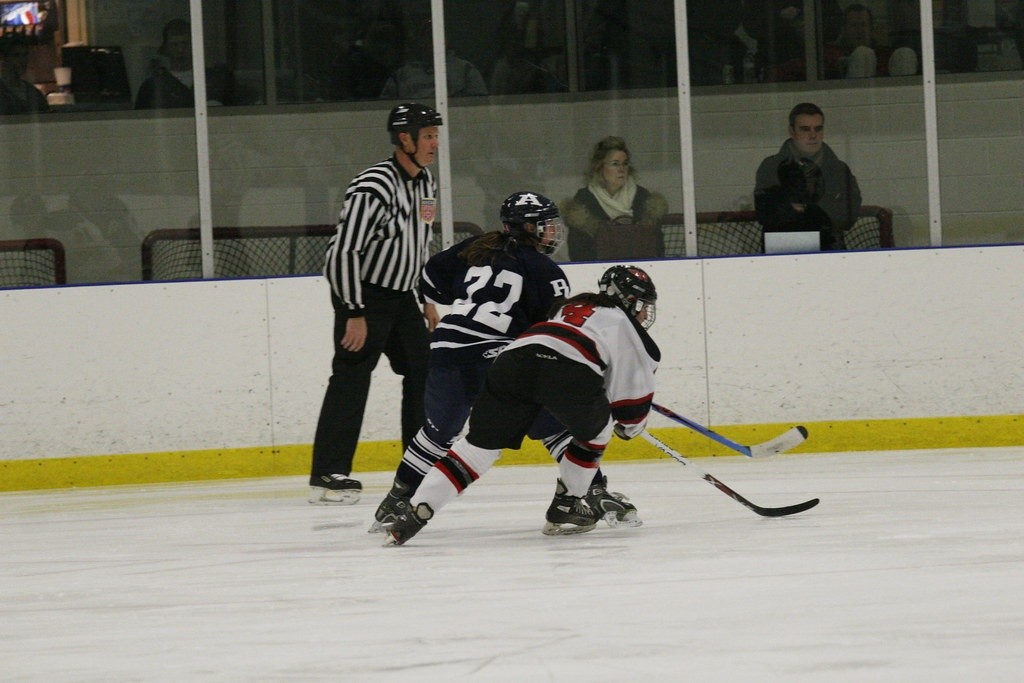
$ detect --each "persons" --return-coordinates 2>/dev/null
[753,104,861,253]
[364,190,661,536]
[0,0,1024,115]
[306,102,444,505]
[557,135,668,261]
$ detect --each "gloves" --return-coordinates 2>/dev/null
[613,423,630,441]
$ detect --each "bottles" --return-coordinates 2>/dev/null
[722,60,735,85]
[744,59,757,83]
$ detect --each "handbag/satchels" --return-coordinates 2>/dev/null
[594,215,657,259]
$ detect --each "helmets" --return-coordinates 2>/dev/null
[501,191,566,257]
[597,265,657,331]
[387,103,444,133]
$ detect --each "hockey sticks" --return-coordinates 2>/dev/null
[650,403,809,460]
[642,430,820,519]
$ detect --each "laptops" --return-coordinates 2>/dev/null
[764,232,820,253]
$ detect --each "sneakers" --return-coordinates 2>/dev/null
[382,502,434,548]
[587,476,643,528]
[367,476,413,534]
[542,477,600,535]
[307,474,362,506]
